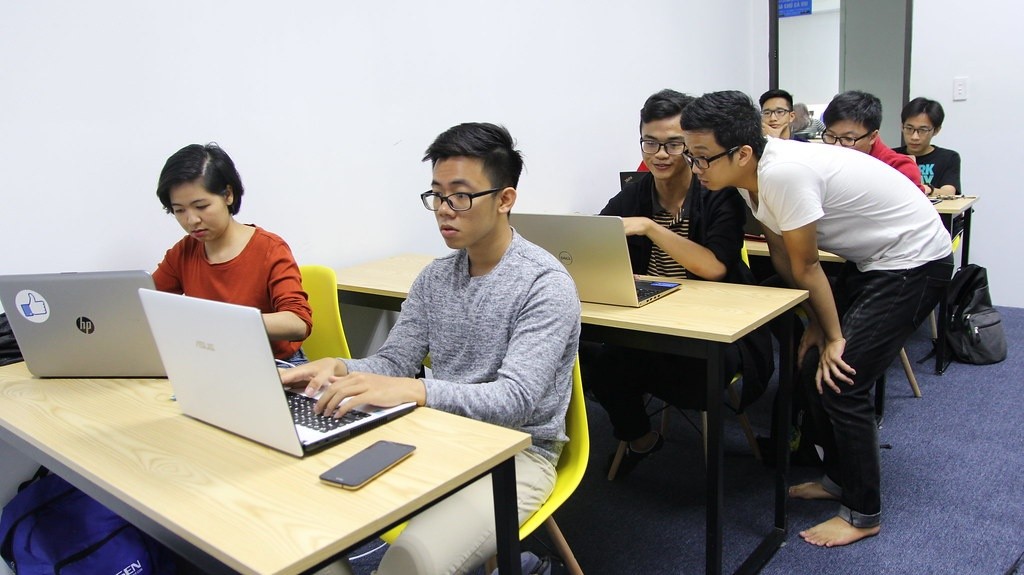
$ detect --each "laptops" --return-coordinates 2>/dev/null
[508,213,682,308]
[138,288,418,458]
[0,270,169,379]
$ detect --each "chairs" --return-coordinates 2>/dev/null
[608,240,767,481]
[380,345,589,575]
[299,266,352,363]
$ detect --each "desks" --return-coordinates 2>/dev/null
[337,252,809,574]
[744,240,885,429]
[0,360,533,575]
[928,194,979,268]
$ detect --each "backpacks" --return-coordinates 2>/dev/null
[0,467,178,575]
[938,266,1007,365]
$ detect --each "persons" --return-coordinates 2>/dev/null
[759,89,825,142]
[149,144,313,370]
[890,98,962,243]
[679,89,954,546]
[823,91,926,195]
[597,91,758,477]
[279,124,583,575]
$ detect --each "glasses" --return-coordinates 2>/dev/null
[902,126,936,137]
[762,108,791,117]
[420,188,502,211]
[821,128,872,148]
[640,138,686,155]
[683,145,740,170]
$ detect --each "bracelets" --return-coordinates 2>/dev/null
[926,183,934,195]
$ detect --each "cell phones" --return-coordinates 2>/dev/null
[930,198,942,204]
[319,441,416,490]
